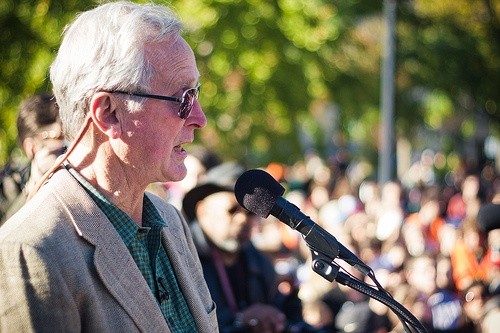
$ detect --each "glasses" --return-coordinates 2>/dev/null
[104,83,202,120]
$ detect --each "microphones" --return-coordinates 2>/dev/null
[234,169,370,277]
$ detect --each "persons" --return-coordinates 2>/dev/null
[1,1,222,333]
[0,95,500,333]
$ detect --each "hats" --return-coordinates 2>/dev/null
[181,160,247,221]
